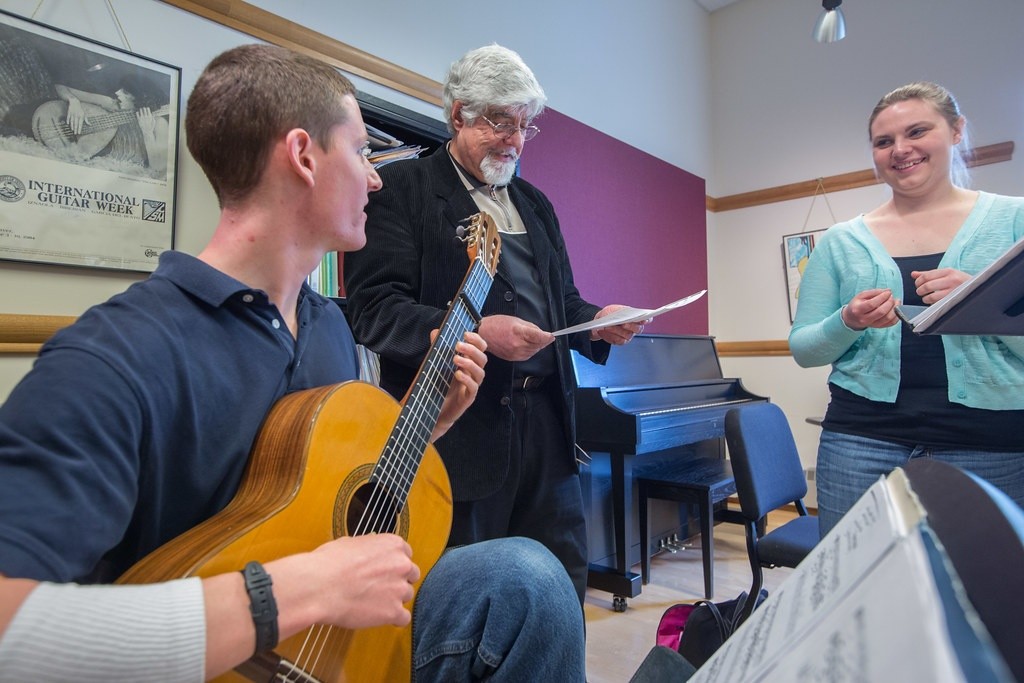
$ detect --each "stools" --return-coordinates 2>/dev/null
[635,457,768,599]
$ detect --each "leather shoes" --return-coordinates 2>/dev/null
[512,375,545,392]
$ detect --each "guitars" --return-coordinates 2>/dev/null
[102,205,503,682]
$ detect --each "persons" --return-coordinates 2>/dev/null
[344,41,655,636]
[55,73,170,175]
[788,80,1024,544]
[0,44,586,683]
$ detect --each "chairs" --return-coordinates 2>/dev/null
[724,402,820,626]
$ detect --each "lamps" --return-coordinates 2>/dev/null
[811,0,847,45]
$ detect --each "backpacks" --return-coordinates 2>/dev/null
[656,588,752,670]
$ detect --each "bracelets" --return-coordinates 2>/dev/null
[241,560,279,656]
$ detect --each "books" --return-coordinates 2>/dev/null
[305,119,429,387]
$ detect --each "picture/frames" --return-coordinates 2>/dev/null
[0,8,182,275]
[783,228,829,324]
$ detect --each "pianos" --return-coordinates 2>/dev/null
[559,330,773,614]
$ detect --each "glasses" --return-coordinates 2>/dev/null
[476,113,540,142]
